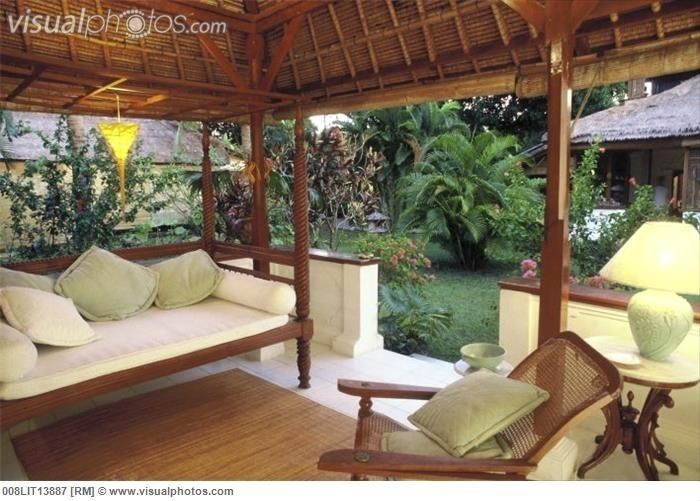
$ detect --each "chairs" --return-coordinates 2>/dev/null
[317,331,624,481]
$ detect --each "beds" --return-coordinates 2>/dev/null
[0,48,314,428]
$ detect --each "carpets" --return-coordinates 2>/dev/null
[11,368,391,481]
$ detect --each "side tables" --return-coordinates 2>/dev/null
[577,335,700,481]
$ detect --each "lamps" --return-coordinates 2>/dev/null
[97,92,140,221]
[598,220,700,361]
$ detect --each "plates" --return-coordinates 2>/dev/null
[605,346,641,368]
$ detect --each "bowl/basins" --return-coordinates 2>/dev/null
[460,342,506,371]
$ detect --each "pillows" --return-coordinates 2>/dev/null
[54,244,160,321]
[0,318,38,384]
[381,430,515,481]
[146,248,224,310]
[0,286,101,347]
[407,367,550,457]
[0,267,57,293]
[211,268,296,315]
